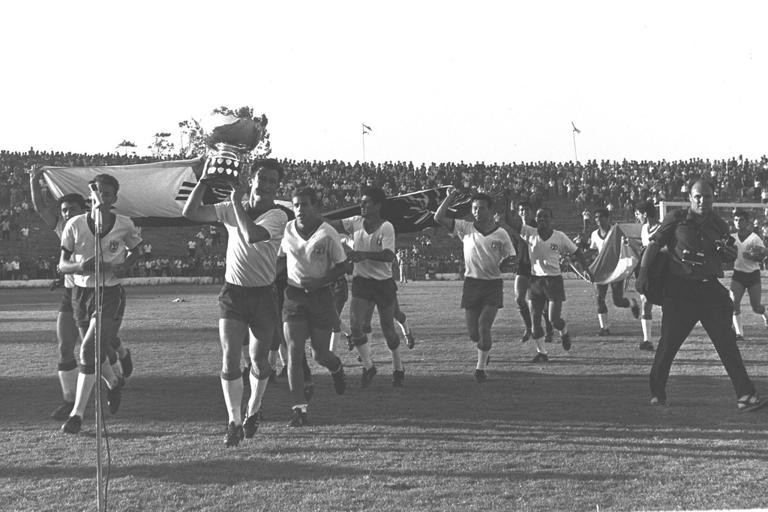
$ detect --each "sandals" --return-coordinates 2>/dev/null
[650,397,665,405]
[737,393,768,412]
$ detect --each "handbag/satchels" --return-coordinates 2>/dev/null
[635,207,688,306]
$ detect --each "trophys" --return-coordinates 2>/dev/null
[198,107,262,189]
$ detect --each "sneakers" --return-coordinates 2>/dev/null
[224,420,244,447]
[268,370,277,384]
[118,375,125,385]
[362,367,377,386]
[242,363,251,384]
[474,368,488,380]
[346,334,354,351]
[118,347,133,377]
[763,319,768,329]
[531,352,549,363]
[596,327,610,336]
[631,298,639,319]
[402,328,414,349]
[281,365,288,376]
[544,329,555,343]
[332,357,347,395]
[304,384,314,402]
[560,331,570,350]
[61,415,82,433]
[640,340,653,349]
[242,405,261,438]
[520,327,531,342]
[486,355,489,365]
[287,408,308,426]
[391,366,405,387]
[735,334,743,340]
[107,381,123,414]
[51,400,74,419]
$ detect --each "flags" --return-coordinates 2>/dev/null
[28,157,230,227]
[362,124,373,135]
[568,222,639,285]
[320,183,475,234]
[572,122,580,133]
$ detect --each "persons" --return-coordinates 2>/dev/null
[320,187,405,389]
[274,206,354,401]
[622,201,668,352]
[60,173,142,435]
[0,147,198,168]
[278,334,288,378]
[358,293,415,362]
[182,223,226,278]
[622,154,768,209]
[347,160,444,204]
[241,319,281,385]
[86,208,133,388]
[585,208,639,336]
[728,211,768,341]
[394,231,465,284]
[634,179,768,413]
[434,186,516,383]
[329,275,355,356]
[274,157,347,209]
[0,168,59,282]
[274,186,348,427]
[132,240,182,277]
[510,200,555,343]
[29,164,126,421]
[559,233,584,273]
[534,160,622,217]
[182,153,288,447]
[444,161,534,201]
[504,199,594,364]
[750,216,768,272]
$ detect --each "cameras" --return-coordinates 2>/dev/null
[681,249,704,267]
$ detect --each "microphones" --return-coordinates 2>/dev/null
[87,180,104,207]
